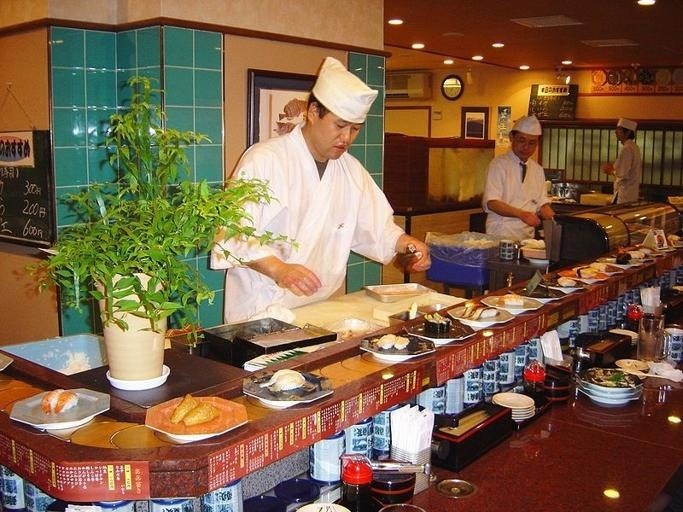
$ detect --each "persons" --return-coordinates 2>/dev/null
[600,116,644,204]
[206,55,433,323]
[480,114,555,245]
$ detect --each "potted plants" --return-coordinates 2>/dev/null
[8,71,302,389]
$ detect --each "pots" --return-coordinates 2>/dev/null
[551,181,581,201]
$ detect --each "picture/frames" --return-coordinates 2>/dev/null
[246,68,319,152]
[460,106,490,141]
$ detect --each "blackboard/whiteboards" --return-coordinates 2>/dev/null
[528,84,578,120]
[0,130,53,248]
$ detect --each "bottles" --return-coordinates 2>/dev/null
[309,402,427,511]
[0,465,246,511]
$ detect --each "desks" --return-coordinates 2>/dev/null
[412,361,683,510]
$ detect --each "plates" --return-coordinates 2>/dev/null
[9,387,110,434]
[295,503,350,511]
[358,237,681,421]
[241,367,334,408]
[143,394,248,445]
[589,67,682,85]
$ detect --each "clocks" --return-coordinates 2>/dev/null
[440,73,464,101]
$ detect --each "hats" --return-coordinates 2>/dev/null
[616,117,639,132]
[312,54,377,124]
[512,115,543,136]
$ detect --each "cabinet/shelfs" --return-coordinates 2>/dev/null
[545,198,682,274]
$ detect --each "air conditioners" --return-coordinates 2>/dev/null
[385,72,434,101]
